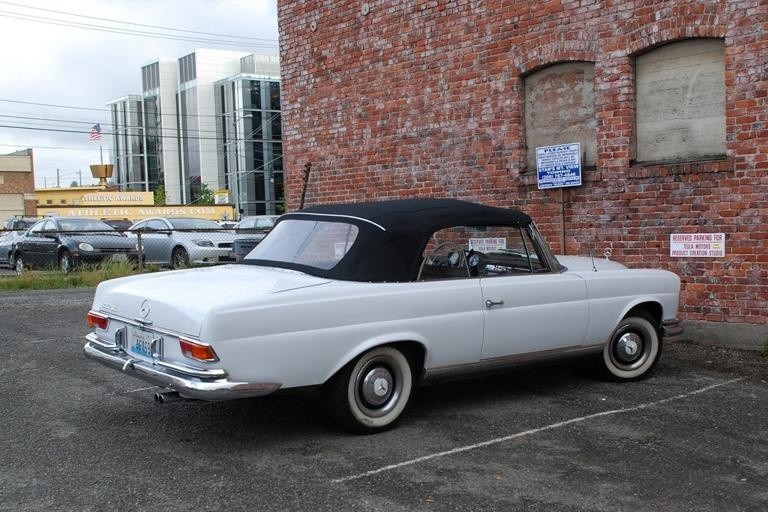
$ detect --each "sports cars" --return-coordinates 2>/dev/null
[80,196,682,434]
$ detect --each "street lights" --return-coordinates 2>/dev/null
[232,114,254,217]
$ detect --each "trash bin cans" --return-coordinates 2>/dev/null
[234,238,262,263]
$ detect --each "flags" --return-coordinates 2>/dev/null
[89,124,101,140]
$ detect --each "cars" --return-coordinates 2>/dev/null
[1,212,282,275]
